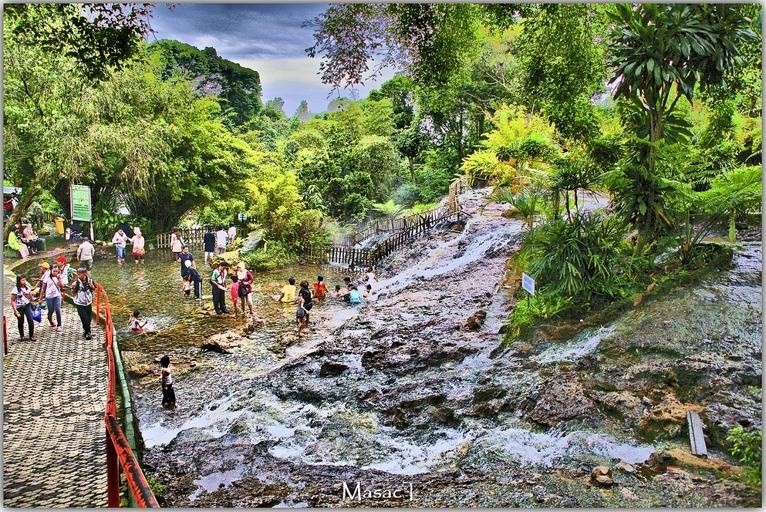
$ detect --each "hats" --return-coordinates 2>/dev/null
[38,255,67,269]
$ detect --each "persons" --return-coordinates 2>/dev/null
[4,191,374,340]
[159,354,177,407]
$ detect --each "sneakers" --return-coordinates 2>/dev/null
[20,335,37,341]
[85,331,92,339]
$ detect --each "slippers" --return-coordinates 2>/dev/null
[48,320,64,332]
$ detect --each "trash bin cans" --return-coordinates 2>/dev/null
[54,217,65,234]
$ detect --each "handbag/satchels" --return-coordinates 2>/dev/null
[238,279,247,298]
